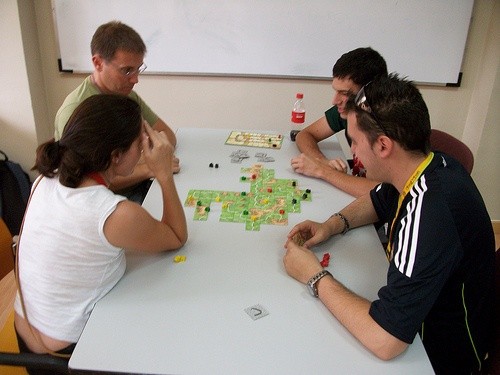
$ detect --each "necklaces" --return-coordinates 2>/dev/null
[88,172,113,190]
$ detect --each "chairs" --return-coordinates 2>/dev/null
[0,218,78,375]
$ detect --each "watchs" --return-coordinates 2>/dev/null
[305,270,333,298]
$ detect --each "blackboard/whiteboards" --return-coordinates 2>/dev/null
[48,0,476,89]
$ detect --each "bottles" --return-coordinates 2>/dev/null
[290,93,306,142]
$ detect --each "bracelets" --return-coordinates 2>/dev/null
[331,213,350,235]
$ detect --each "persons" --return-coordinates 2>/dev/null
[15,93,187,375]
[283,73,500,375]
[290,47,388,199]
[54,22,181,206]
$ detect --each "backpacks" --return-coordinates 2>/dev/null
[0,150,32,235]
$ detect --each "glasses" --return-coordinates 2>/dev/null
[354,85,389,138]
[109,60,147,78]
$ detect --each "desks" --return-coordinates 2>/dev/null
[68,127,436,375]
